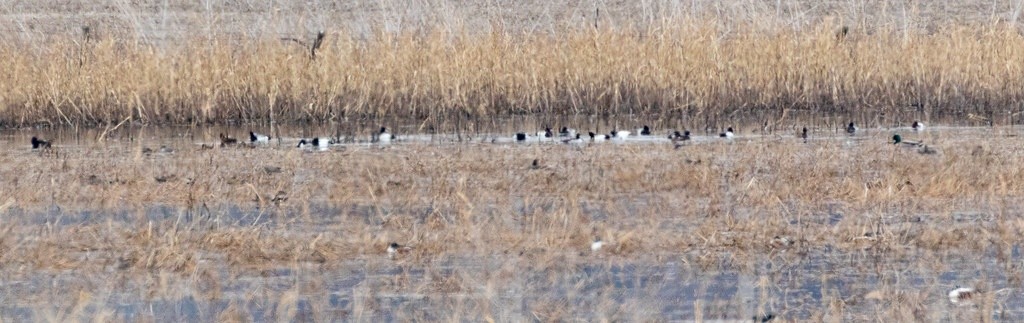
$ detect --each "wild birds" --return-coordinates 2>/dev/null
[276,30,326,61]
[32,120,936,261]
[948,284,980,305]
[751,313,780,323]
